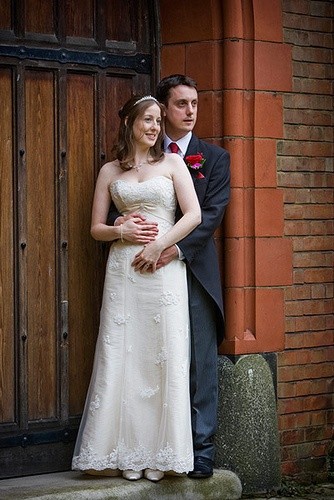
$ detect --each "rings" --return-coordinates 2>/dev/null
[145,264,150,268]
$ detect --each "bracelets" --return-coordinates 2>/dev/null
[120,224,124,243]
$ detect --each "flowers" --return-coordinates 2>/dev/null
[185,153,206,183]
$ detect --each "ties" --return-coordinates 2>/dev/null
[169,143,179,154]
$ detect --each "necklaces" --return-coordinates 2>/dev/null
[128,159,150,171]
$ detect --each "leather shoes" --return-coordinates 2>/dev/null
[144,469,164,480]
[122,471,142,480]
[187,456,213,478]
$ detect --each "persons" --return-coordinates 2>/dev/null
[106,74,231,480]
[72,96,201,480]
